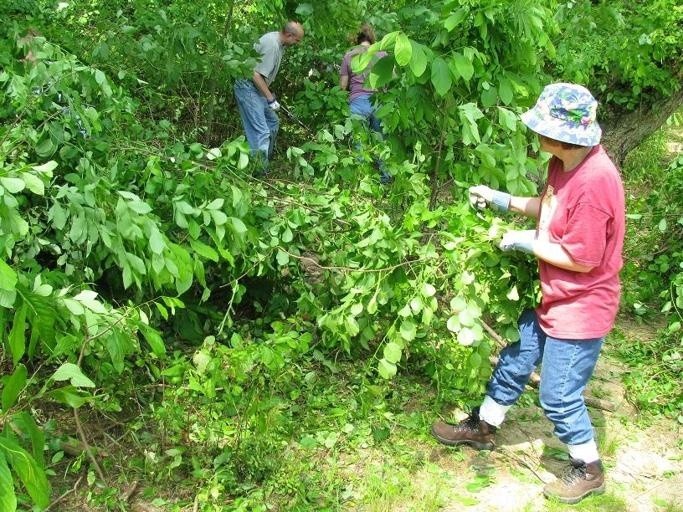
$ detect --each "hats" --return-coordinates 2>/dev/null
[519,82,603,147]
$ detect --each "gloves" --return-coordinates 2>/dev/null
[267,98,281,112]
[499,230,537,256]
[468,184,511,214]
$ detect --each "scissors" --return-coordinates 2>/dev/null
[280,105,308,132]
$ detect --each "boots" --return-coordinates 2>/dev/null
[430,405,497,450]
[542,452,606,504]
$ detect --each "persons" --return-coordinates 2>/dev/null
[426,80,628,507]
[337,28,397,186]
[230,21,305,162]
